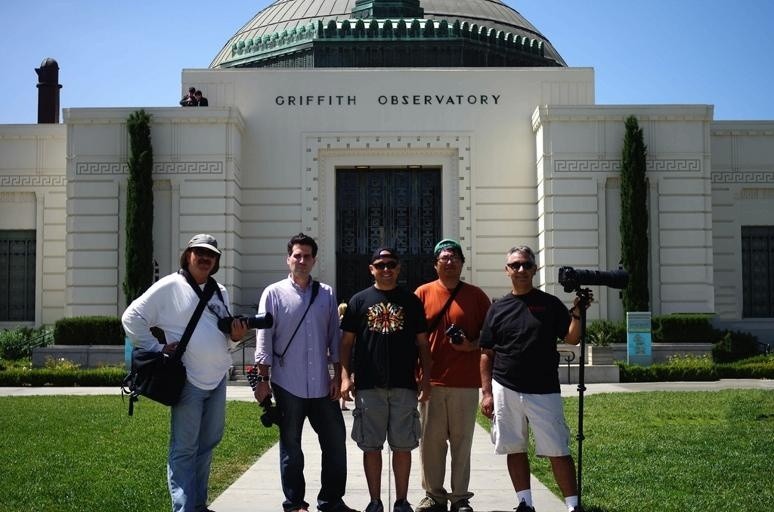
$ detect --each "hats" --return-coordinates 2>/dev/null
[370,247,398,263]
[435,239,462,251]
[188,234,220,254]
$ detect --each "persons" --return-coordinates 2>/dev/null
[253,232,360,511]
[180,87,200,107]
[194,89,208,106]
[414,238,492,512]
[339,246,431,511]
[118,232,252,512]
[480,244,596,510]
[338,301,348,412]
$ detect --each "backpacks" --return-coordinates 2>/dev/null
[124,346,186,406]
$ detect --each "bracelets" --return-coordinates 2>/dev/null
[572,311,583,323]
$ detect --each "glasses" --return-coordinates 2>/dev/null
[438,257,463,262]
[373,262,397,270]
[508,261,534,268]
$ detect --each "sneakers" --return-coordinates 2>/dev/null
[392,499,414,512]
[363,500,384,512]
[417,496,448,512]
[450,499,474,512]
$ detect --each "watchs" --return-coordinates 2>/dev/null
[256,375,270,383]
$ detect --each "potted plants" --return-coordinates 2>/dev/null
[587,330,614,365]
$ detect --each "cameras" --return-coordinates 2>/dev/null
[218,312,273,333]
[447,324,467,346]
[260,403,286,427]
